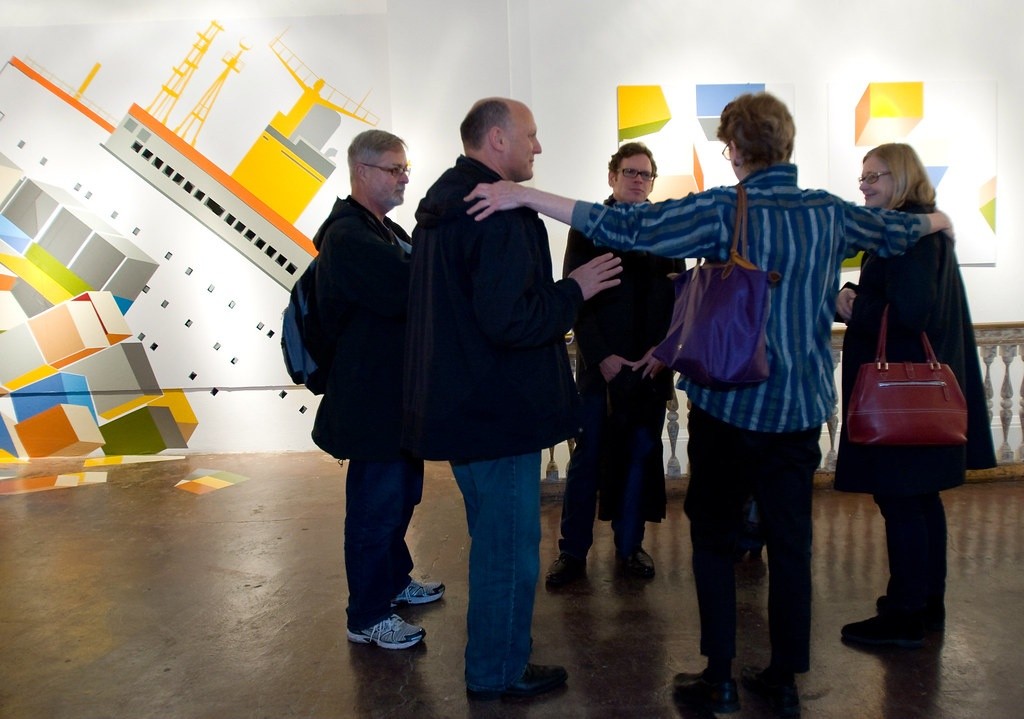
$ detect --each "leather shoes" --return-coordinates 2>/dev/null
[466,664,568,701]
[741,666,801,715]
[673,674,741,713]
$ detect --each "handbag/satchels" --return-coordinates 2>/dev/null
[652,185,769,391]
[846,303,968,446]
[598,384,666,523]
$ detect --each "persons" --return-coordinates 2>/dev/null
[836,142,1000,648]
[402,100,623,702]
[463,83,956,711]
[313,129,446,650]
[545,141,687,583]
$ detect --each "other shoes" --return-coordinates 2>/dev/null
[736,558,767,582]
[877,596,887,611]
[841,617,936,651]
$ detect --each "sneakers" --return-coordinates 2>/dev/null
[347,614,427,650]
[616,549,655,578]
[392,580,445,605]
[546,553,586,590]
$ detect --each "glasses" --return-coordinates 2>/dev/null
[722,144,730,159]
[859,172,890,184]
[361,163,408,177]
[616,169,652,181]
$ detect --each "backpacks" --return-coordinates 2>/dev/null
[281,260,327,395]
